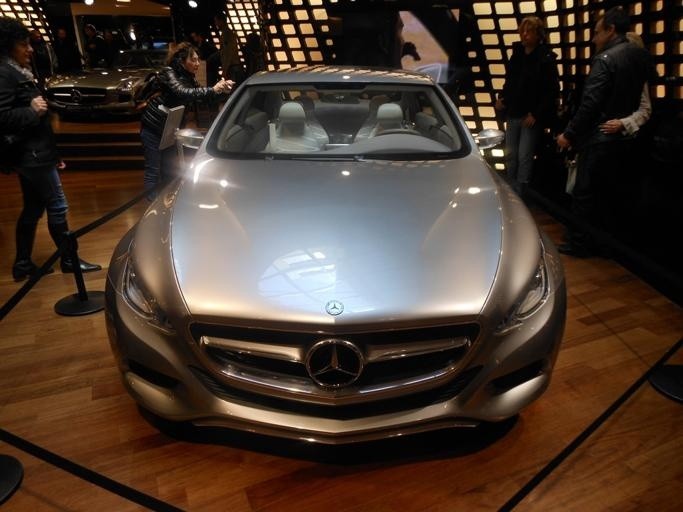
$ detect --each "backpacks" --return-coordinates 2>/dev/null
[132,68,176,111]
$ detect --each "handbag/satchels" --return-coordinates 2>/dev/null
[0,129,24,175]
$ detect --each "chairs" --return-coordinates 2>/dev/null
[353,94,403,143]
[294,97,330,151]
[264,101,319,153]
[368,102,410,139]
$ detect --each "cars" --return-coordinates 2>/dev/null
[44,50,169,116]
[105,65,567,447]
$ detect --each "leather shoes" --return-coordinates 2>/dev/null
[553,241,592,258]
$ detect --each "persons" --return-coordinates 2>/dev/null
[0,15,101,282]
[600,30,654,138]
[554,8,648,261]
[494,17,559,203]
[137,45,237,201]
[27,22,268,114]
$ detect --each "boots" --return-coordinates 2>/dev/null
[48,223,101,272]
[12,221,54,282]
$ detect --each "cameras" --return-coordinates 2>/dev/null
[225,81,236,90]
